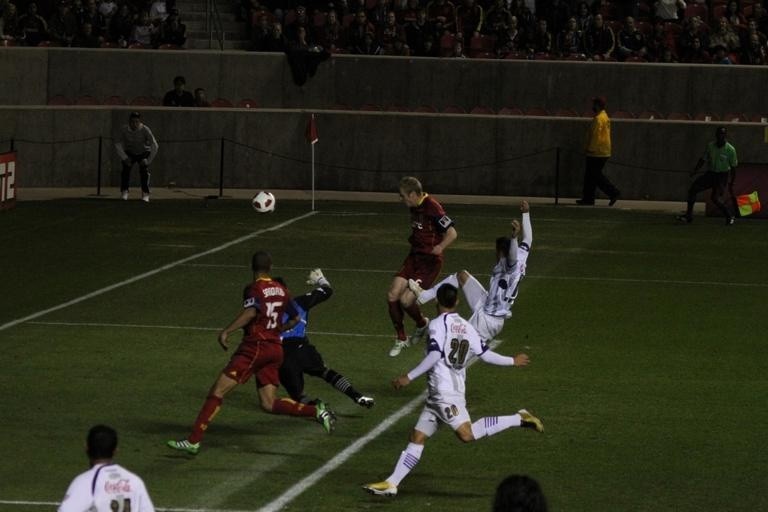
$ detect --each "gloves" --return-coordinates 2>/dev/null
[305,268,331,286]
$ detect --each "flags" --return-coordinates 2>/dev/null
[736,190,761,217]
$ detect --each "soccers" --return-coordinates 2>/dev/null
[252,191,275,214]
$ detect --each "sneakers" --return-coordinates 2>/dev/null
[387,279,429,357]
[360,481,399,498]
[518,408,544,434]
[166,395,377,456]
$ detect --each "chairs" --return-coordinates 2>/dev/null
[0,39,197,50]
[610,109,768,123]
[48,95,260,107]
[242,0,768,63]
[327,103,595,118]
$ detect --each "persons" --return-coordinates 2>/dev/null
[194,88,211,105]
[387,176,458,357]
[167,251,334,454]
[241,2,766,65]
[674,125,738,226]
[271,267,375,427]
[575,95,622,206]
[492,474,548,511]
[2,2,186,51]
[163,75,195,106]
[361,286,544,497]
[56,424,156,512]
[113,110,158,202]
[407,200,533,359]
